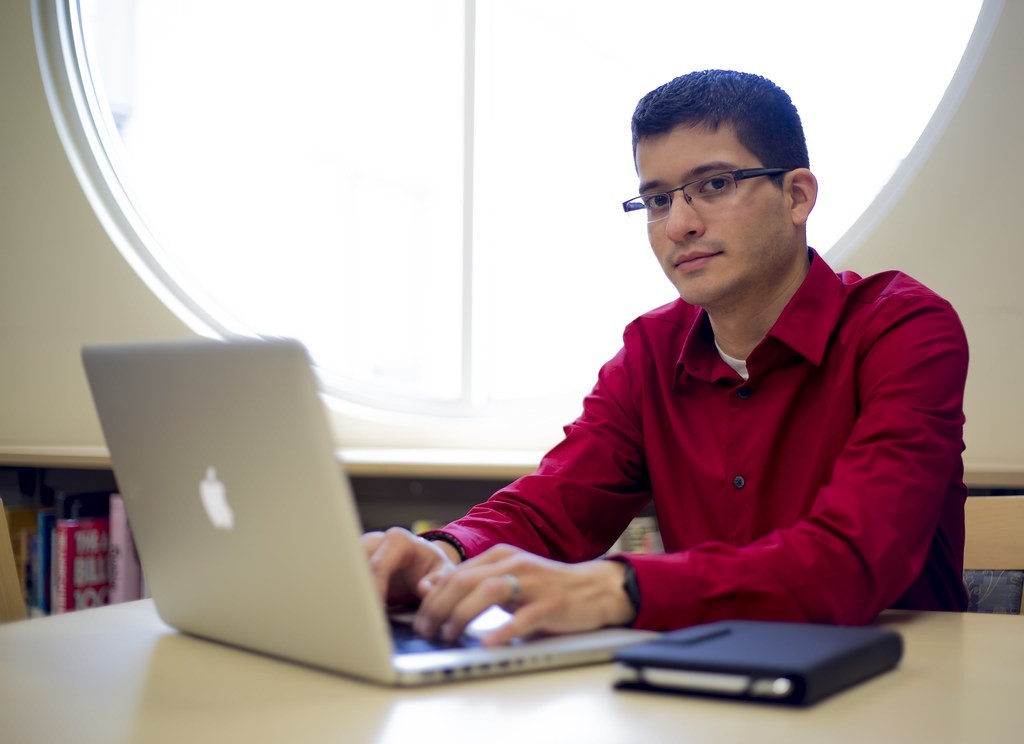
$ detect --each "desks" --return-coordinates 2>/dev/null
[0,595,1024,744]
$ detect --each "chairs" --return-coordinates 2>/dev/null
[963,496,1024,615]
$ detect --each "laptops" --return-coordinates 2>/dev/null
[80,337,661,686]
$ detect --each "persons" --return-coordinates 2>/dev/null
[358,69,969,649]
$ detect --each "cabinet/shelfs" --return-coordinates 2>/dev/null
[0,443,1024,623]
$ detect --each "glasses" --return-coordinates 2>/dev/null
[622,166,787,226]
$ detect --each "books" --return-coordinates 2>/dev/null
[598,516,666,555]
[613,617,904,707]
[12,489,142,622]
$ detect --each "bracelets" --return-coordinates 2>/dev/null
[505,573,520,603]
